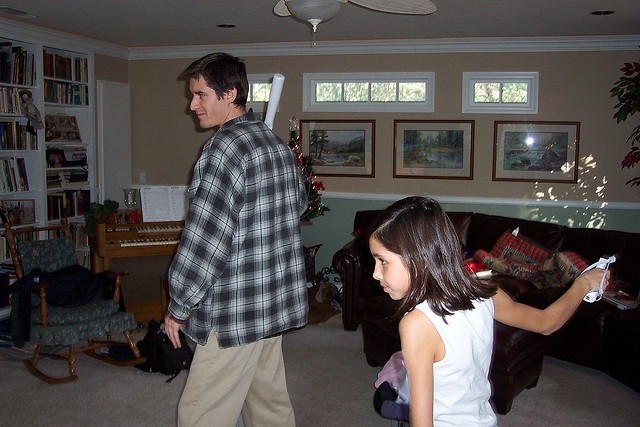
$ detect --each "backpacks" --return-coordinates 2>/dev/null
[142,318,194,383]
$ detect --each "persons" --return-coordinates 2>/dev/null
[19,88,32,112]
[162,50,311,426]
[367,194,612,426]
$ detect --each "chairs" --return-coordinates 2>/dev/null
[3,215,148,386]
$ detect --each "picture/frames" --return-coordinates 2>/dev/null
[491,119,580,185]
[392,117,476,181]
[296,117,377,179]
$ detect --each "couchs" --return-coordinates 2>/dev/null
[329,205,640,396]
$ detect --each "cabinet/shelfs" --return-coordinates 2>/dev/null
[1,36,107,322]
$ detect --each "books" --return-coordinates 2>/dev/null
[42,49,91,83]
[60,144,88,167]
[47,189,90,221]
[74,248,91,270]
[137,184,191,224]
[41,76,89,106]
[1,117,38,150]
[0,86,20,114]
[0,40,38,86]
[0,230,40,261]
[48,222,90,249]
[0,263,19,309]
[46,168,90,189]
[0,156,30,194]
